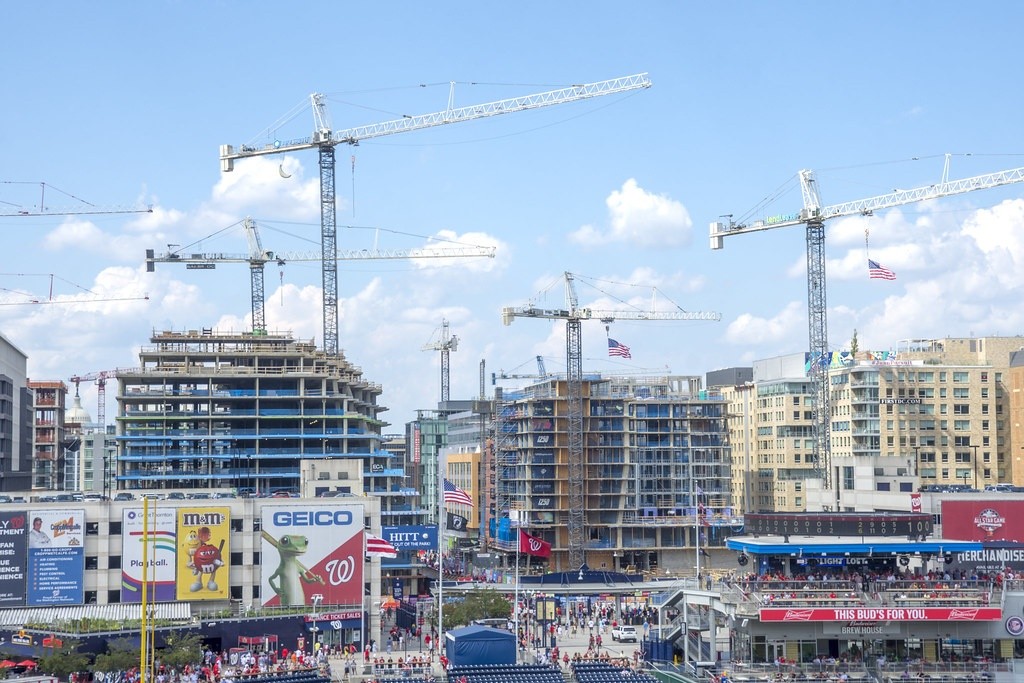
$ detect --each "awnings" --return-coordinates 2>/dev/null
[303,618,367,632]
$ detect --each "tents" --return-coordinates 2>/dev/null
[445,625,516,667]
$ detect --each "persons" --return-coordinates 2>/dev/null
[1,544,1024,682]
[27,516,52,549]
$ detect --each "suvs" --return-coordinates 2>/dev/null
[612,624,638,643]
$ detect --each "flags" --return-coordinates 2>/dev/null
[443,477,476,508]
[518,527,553,560]
[446,511,469,532]
[608,338,632,359]
[363,531,399,560]
[868,257,896,282]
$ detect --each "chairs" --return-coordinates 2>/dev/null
[447,662,566,683]
[571,662,664,683]
[374,677,438,683]
[224,670,331,683]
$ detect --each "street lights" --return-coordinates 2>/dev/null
[144,604,159,683]
[310,593,324,663]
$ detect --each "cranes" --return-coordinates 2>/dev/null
[1,180,155,220]
[708,149,1024,493]
[420,316,462,403]
[0,272,152,307]
[501,270,723,577]
[219,70,655,359]
[144,214,499,339]
[69,366,160,436]
